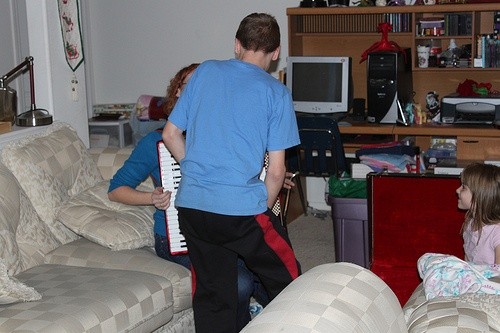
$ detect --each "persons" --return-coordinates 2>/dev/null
[162,13,301,333]
[108,64,294,333]
[456,163,500,265]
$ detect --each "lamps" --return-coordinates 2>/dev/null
[0,57,53,126]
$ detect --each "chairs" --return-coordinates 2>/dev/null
[282,116,348,217]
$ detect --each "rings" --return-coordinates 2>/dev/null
[160,203,161,206]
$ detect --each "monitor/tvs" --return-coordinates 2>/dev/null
[287,56,353,120]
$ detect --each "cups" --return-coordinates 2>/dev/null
[417,44,430,68]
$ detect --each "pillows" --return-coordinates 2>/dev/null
[55,179,156,251]
[0,261,41,305]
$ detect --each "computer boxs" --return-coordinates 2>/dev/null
[366,47,413,123]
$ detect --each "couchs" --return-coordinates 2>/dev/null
[0,122,500,333]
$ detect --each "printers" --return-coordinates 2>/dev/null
[440,94,500,129]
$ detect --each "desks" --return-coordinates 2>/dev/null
[425,160,484,173]
[335,117,500,163]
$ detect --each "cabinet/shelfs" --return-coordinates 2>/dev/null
[89,117,133,150]
[286,2,500,113]
